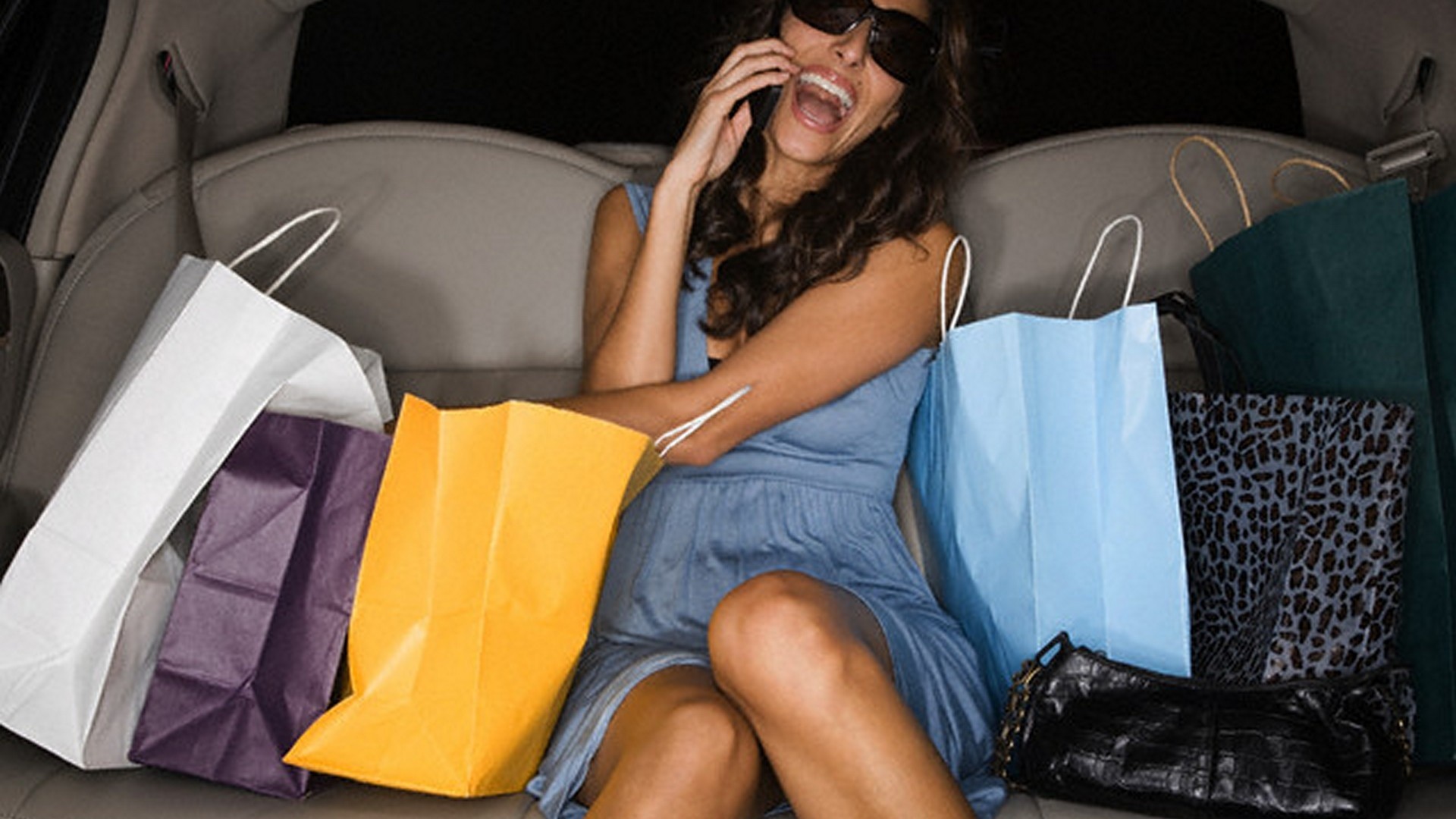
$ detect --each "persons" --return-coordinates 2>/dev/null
[533,3,976,817]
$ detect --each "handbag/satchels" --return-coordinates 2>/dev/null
[283,390,753,794]
[129,410,392,811]
[995,633,1398,819]
[1153,292,1414,681]
[0,205,392,770]
[1170,136,1454,779]
[910,213,1191,711]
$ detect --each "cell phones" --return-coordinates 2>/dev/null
[747,26,782,129]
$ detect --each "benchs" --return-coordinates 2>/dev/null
[0,115,1388,817]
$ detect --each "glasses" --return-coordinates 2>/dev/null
[790,0,938,85]
